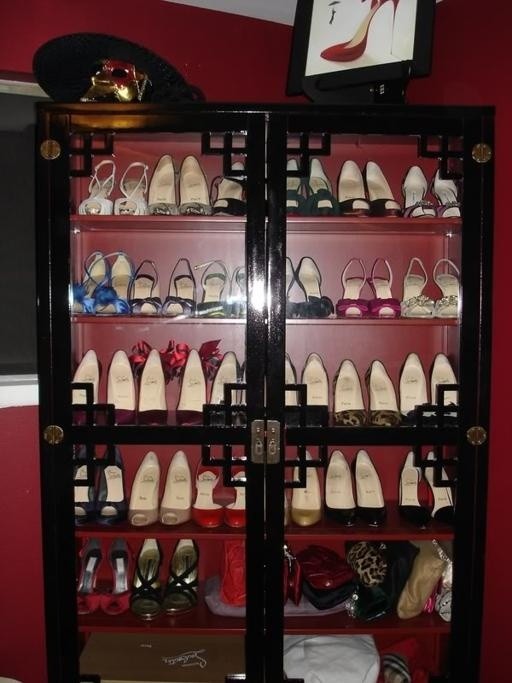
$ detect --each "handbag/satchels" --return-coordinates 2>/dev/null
[204,538,453,623]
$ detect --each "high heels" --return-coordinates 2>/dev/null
[321,0,400,62]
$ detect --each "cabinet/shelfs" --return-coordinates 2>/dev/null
[36,103,493,682]
